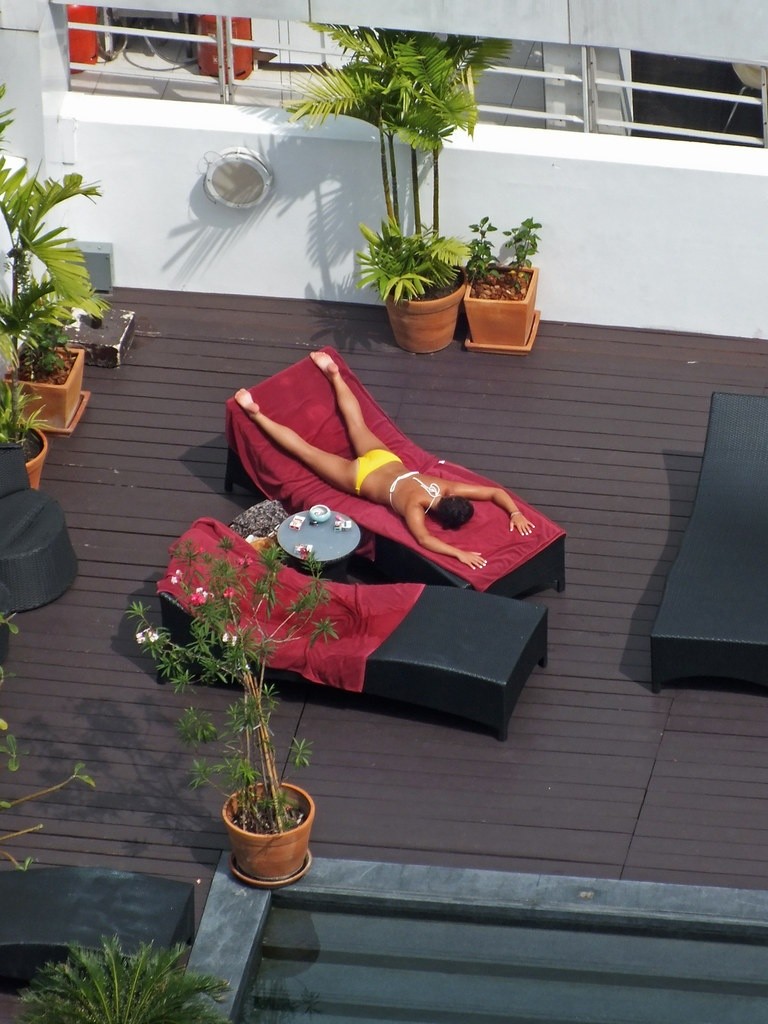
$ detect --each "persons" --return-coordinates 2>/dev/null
[233,351,535,569]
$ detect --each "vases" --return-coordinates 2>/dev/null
[222,782,315,881]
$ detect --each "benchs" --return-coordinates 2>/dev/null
[0,863,195,986]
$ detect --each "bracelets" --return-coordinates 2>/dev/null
[510,511,521,517]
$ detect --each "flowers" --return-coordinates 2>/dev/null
[121,535,339,829]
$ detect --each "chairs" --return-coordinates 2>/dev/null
[723,61,762,130]
[156,517,549,741]
[648,392,768,695]
[224,344,569,599]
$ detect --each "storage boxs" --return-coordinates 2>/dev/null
[295,543,315,556]
[289,515,306,531]
[333,521,342,532]
[343,521,352,531]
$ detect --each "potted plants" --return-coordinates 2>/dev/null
[0,380,57,490]
[0,82,113,427]
[463,217,543,344]
[284,19,513,353]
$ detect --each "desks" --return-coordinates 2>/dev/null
[276,510,362,585]
